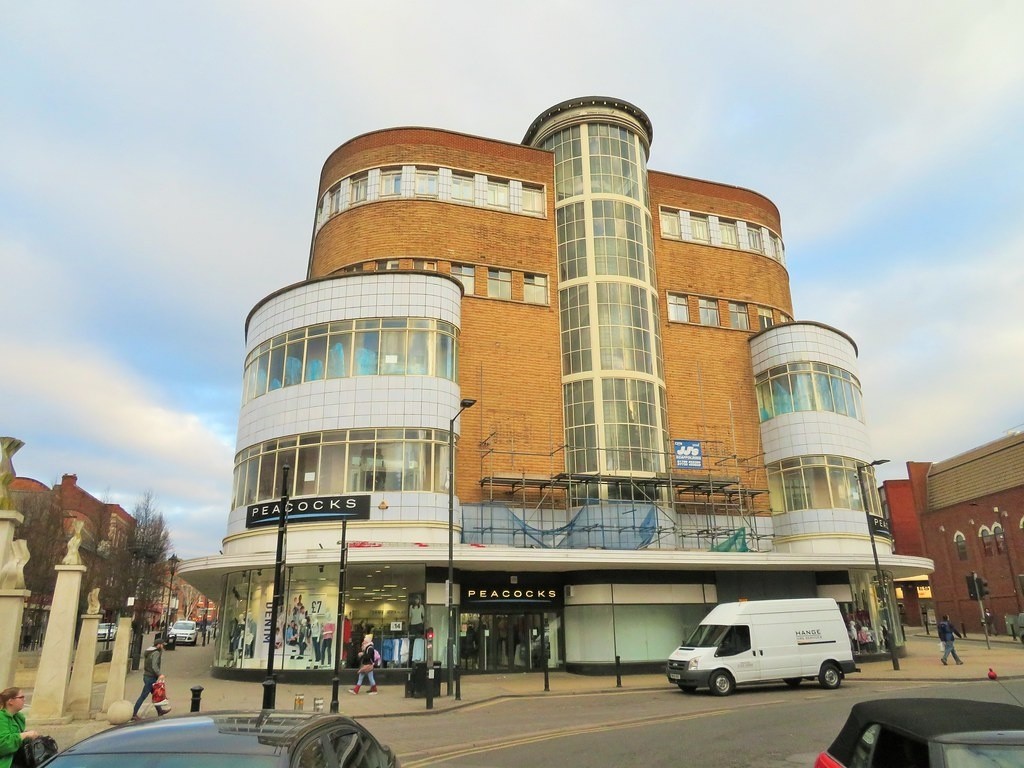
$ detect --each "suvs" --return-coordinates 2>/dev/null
[167,621,200,646]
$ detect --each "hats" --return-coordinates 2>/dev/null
[414,594,422,601]
[154,638,167,646]
[364,633,373,639]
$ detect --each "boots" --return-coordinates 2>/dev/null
[348,685,360,695]
[367,685,377,693]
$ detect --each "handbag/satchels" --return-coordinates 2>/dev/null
[14,736,58,768]
[152,682,168,706]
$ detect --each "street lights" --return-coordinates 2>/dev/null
[856,458,907,671]
[445,395,477,698]
[969,502,1021,613]
[163,553,179,642]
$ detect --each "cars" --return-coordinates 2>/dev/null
[811,694,1023,767]
[38,710,399,767]
[96,623,118,642]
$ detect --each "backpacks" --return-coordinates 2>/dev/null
[367,646,382,667]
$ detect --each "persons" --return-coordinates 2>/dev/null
[848,617,889,651]
[230,614,246,661]
[408,594,425,635]
[307,612,323,667]
[129,638,172,722]
[348,632,379,695]
[151,619,166,632]
[0,686,37,768]
[242,612,257,659]
[984,607,999,637]
[317,612,335,668]
[275,594,312,662]
[937,614,964,666]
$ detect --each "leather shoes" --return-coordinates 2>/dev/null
[131,715,145,722]
[158,708,172,718]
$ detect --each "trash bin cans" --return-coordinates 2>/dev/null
[167,634,177,650]
[411,661,442,699]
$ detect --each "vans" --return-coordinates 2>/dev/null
[661,598,862,696]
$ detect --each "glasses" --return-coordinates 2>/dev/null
[12,694,25,702]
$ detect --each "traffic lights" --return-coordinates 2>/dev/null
[976,576,990,599]
